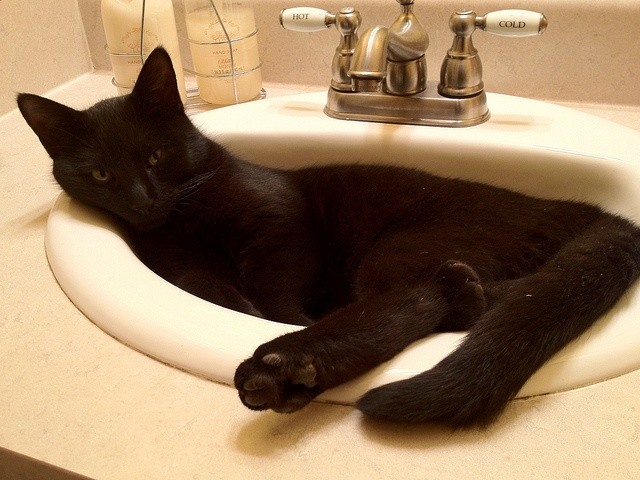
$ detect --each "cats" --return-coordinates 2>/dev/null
[14,44,640,436]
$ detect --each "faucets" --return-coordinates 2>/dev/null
[347,0,430,96]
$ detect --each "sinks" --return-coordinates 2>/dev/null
[44,91,639,406]
